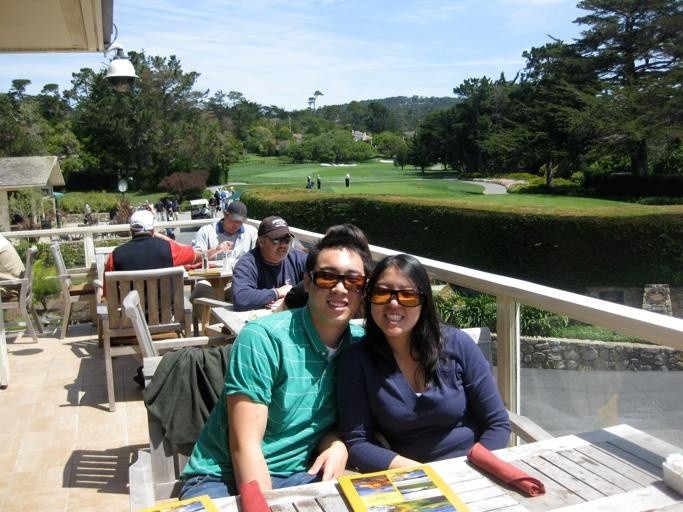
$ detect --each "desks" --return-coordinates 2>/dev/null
[182,258,237,336]
[211,305,366,340]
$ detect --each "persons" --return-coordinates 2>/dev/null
[103,210,197,323]
[84,201,92,224]
[274,224,380,312]
[345,171,352,187]
[336,253,511,468]
[0,232,27,301]
[110,186,236,239]
[316,174,322,189]
[306,176,312,189]
[231,215,305,311]
[193,199,260,306]
[176,239,376,501]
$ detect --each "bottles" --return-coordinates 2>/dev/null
[201,250,209,271]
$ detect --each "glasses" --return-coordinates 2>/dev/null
[366,287,424,307]
[308,271,367,293]
[272,236,291,244]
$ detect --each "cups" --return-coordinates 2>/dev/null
[222,250,237,272]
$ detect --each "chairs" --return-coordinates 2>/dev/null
[142,356,196,502]
[95,247,115,282]
[0,247,44,341]
[193,294,235,333]
[121,286,208,384]
[50,242,98,338]
[462,324,555,443]
[101,266,193,410]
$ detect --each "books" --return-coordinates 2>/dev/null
[339,466,469,512]
[136,495,218,512]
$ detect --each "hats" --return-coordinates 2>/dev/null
[130,210,154,231]
[227,202,247,223]
[258,216,295,240]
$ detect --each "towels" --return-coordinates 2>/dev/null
[240,481,270,512]
[467,442,545,496]
